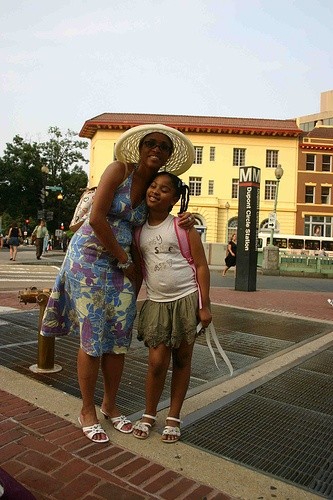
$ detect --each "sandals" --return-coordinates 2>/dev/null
[133,412,159,439]
[161,416,183,443]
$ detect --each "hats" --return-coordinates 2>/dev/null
[114,124,195,176]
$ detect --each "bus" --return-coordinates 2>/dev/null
[254,232,333,279]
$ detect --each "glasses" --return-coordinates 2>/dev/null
[142,140,173,152]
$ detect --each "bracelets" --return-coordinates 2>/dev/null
[117,253,133,269]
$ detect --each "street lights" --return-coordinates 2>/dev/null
[267,165,283,246]
[41,158,49,221]
[224,201,230,259]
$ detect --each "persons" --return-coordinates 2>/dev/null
[61,232,70,252]
[40,124,195,443]
[130,172,212,444]
[6,223,22,261]
[314,247,324,260]
[32,219,48,260]
[222,234,237,277]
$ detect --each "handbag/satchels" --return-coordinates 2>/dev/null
[32,235,36,241]
[69,186,97,232]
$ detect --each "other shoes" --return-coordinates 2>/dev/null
[10,257,13,260]
[327,298,333,306]
[37,256,41,260]
[223,271,226,276]
[13,258,15,261]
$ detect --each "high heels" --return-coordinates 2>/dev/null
[78,417,109,443]
[100,408,133,433]
